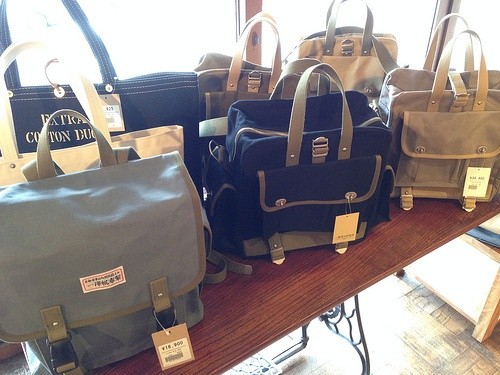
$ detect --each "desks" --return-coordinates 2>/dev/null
[0,195,500,374]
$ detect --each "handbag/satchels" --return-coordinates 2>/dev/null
[376,12,500,212]
[203,58,397,265]
[0,109,252,375]
[0,36,185,190]
[190,11,284,122]
[0,0,202,212]
[296,0,398,106]
